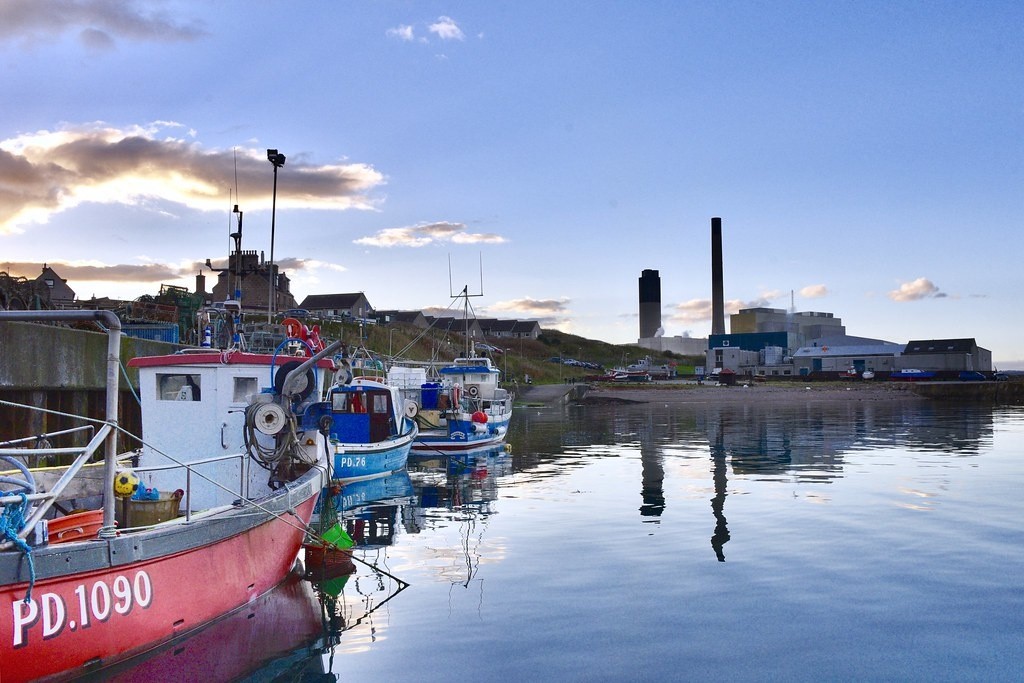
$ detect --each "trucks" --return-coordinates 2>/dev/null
[959,371,986,382]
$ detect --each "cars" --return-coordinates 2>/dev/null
[492,346,504,353]
[547,357,598,369]
[277,309,316,319]
[324,315,363,323]
[994,373,1008,381]
[475,344,495,352]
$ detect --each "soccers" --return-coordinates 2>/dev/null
[115,469,139,498]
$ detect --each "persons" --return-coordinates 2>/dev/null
[479,351,487,366]
[564,376,575,385]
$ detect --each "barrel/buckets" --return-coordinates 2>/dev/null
[114,489,184,532]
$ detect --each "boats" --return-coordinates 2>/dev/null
[889,368,938,382]
[604,354,677,382]
[66,555,345,683]
[325,323,418,484]
[839,365,862,382]
[0,148,336,683]
[387,250,513,450]
[862,371,875,380]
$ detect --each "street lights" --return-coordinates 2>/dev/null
[626,353,630,370]
[268,148,286,324]
[560,351,566,379]
[505,348,511,382]
[579,348,582,361]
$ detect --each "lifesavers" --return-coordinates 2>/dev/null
[453,383,462,408]
[281,318,302,338]
[354,376,383,382]
